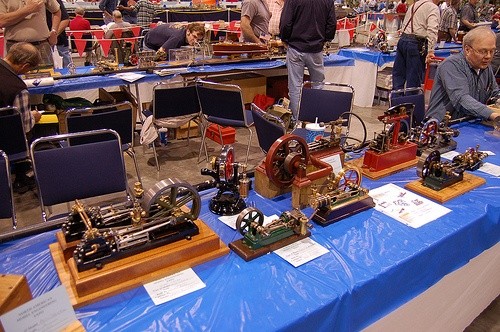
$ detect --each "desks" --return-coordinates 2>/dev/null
[0,115,500,332]
[338,41,464,108]
[16,52,353,146]
[369,12,403,33]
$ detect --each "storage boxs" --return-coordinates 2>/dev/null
[205,122,236,145]
[26,103,59,146]
[169,121,201,139]
[266,104,293,132]
[99,85,136,129]
[200,72,266,104]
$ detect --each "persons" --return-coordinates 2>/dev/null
[423,26,500,122]
[392,0,441,97]
[396,0,407,30]
[279,0,337,128]
[241,0,284,43]
[143,23,205,61]
[45,0,73,67]
[99,0,156,29]
[435,0,465,44]
[105,9,131,38]
[0,41,41,193]
[0,0,61,69]
[456,0,483,38]
[70,7,91,30]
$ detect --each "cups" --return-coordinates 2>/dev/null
[305,124,325,143]
[159,128,168,146]
[440,39,446,46]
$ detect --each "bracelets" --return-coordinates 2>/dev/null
[52,29,58,34]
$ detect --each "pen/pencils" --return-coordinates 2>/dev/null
[35,106,38,112]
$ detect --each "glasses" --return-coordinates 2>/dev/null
[466,44,498,56]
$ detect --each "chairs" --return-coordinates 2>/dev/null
[140,29,151,48]
[0,80,425,231]
